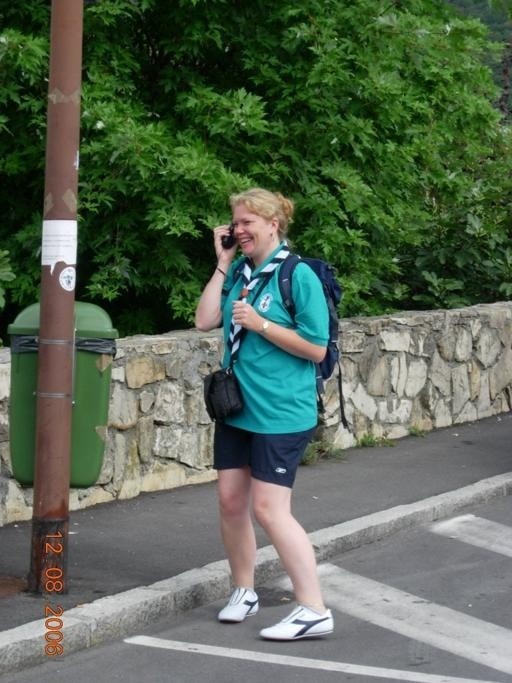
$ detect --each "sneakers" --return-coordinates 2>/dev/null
[217,586,259,623]
[258,603,335,639]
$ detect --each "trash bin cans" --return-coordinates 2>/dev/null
[7,300,120,487]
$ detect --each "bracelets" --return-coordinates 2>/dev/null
[194,187,342,641]
[216,266,226,276]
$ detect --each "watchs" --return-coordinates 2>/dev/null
[258,320,270,336]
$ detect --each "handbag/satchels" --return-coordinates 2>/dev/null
[203,368,244,422]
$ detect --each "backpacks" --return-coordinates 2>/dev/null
[278,254,342,380]
